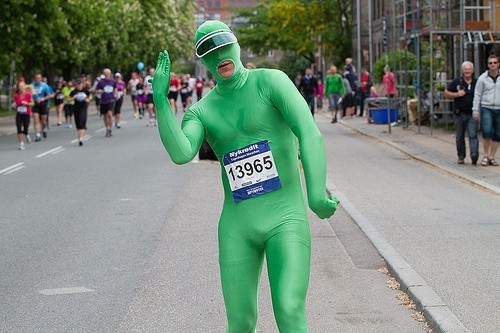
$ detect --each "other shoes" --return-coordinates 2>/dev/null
[57,121,73,128]
[133,111,156,126]
[331,112,363,123]
[472,153,479,165]
[458,158,465,165]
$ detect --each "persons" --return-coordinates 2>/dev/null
[367,82,380,97]
[148,21,340,333]
[358,68,373,117]
[246,63,255,68]
[168,72,217,115]
[340,58,358,120]
[323,65,346,123]
[444,61,478,165]
[30,73,50,141]
[12,81,35,150]
[294,73,302,88]
[298,69,318,119]
[128,68,158,126]
[379,65,397,98]
[314,79,323,109]
[92,68,126,137]
[41,77,55,131]
[54,74,93,146]
[472,54,500,166]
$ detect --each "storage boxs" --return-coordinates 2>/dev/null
[370,108,398,124]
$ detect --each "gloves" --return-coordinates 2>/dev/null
[309,193,340,219]
[148,50,171,97]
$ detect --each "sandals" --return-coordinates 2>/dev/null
[489,158,499,166]
[482,156,489,167]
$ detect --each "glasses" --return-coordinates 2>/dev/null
[488,62,498,65]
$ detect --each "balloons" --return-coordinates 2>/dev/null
[137,62,144,70]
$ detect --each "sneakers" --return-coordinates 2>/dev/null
[19,142,25,150]
[106,129,113,138]
[116,122,122,129]
[79,140,83,146]
[43,128,47,138]
[34,136,41,141]
[27,136,32,143]
